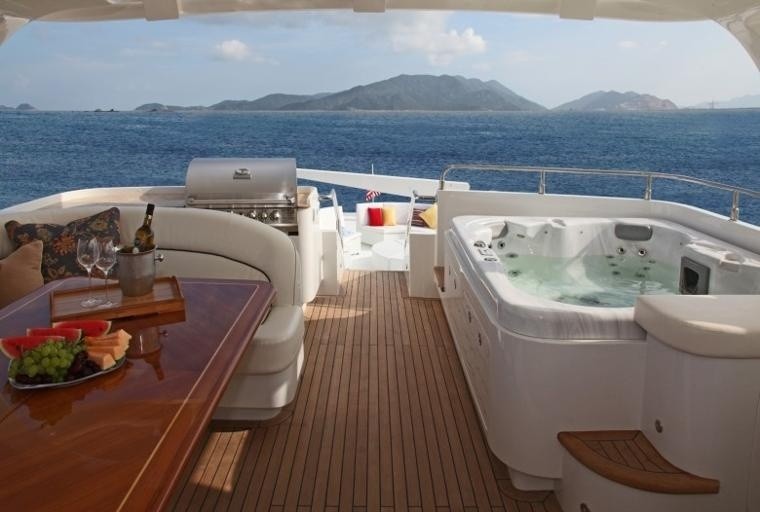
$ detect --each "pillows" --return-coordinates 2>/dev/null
[5,208,119,284]
[0,240,44,308]
[382,207,397,226]
[367,206,383,226]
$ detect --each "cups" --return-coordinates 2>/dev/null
[118,244,164,296]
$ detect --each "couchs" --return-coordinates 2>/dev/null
[0,206,305,421]
[356,202,432,245]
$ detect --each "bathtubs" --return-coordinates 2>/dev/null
[447,214,760,341]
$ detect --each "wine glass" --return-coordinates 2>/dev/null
[77,236,116,308]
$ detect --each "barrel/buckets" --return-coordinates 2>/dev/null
[116,248,157,296]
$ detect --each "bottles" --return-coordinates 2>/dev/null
[131,203,154,254]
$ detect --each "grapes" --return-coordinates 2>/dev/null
[8,340,98,385]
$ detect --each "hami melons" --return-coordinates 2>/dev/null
[82,328,132,370]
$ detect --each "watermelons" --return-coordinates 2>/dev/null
[0,319,112,361]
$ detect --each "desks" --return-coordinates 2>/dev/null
[0,276,278,512]
